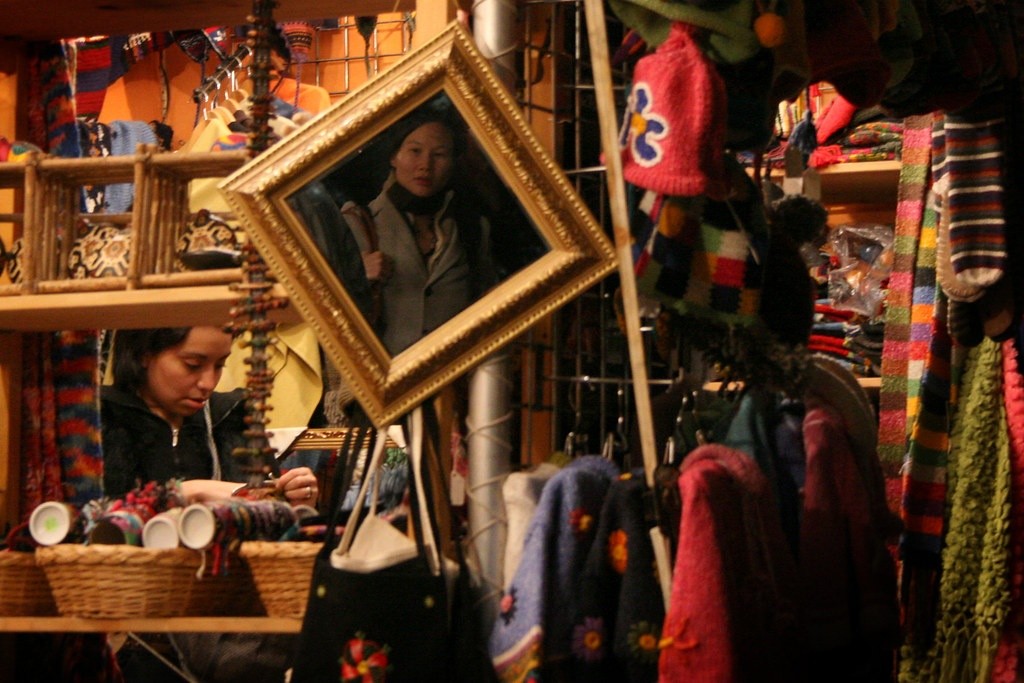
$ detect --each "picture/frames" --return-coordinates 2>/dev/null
[216,22,620,431]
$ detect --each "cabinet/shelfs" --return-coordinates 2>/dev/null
[0,281,299,632]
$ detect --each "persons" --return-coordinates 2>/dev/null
[100,325,320,514]
[340,115,498,359]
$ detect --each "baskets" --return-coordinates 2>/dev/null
[33,541,325,620]
[0,547,58,616]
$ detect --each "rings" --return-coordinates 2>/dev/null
[305,485,313,499]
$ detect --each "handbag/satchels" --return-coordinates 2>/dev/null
[287,398,504,683]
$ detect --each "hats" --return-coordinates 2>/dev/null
[607,0,1024,352]
